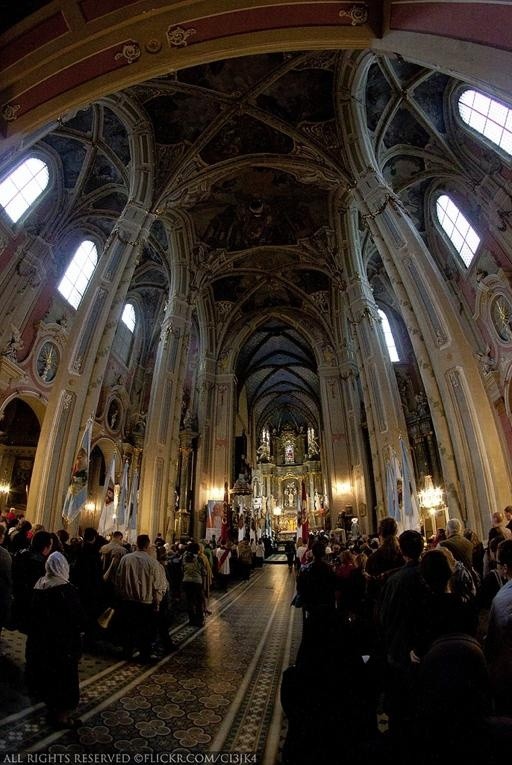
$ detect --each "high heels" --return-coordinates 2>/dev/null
[204,609,212,616]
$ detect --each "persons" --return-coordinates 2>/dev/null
[11,551,93,730]
[279,510,512,765]
[0,507,278,669]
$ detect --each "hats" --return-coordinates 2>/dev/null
[295,544,309,562]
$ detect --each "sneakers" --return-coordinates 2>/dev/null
[53,717,83,731]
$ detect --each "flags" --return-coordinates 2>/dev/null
[51,417,139,544]
[385,436,422,538]
